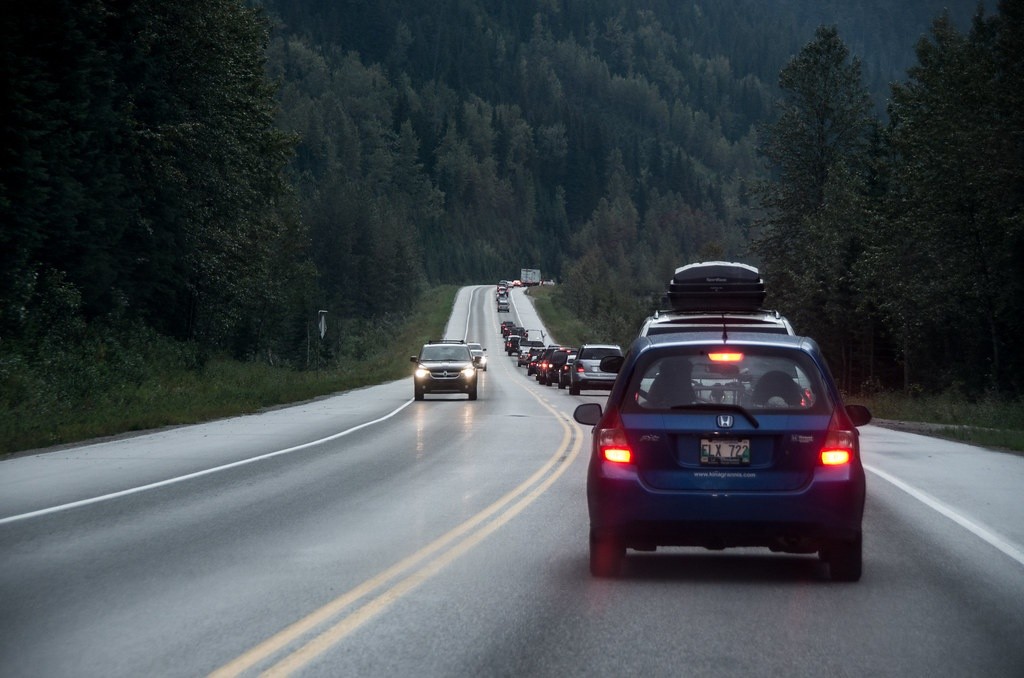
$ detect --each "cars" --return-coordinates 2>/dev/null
[496,278,523,313]
[465,342,488,371]
[572,330,872,583]
[500,320,579,390]
[539,278,555,288]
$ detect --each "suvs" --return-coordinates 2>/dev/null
[567,343,625,395]
[599,259,817,412]
[410,339,478,401]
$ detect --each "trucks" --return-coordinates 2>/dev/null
[521,269,541,288]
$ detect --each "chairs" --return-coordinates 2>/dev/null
[753,370,811,406]
[647,373,696,406]
